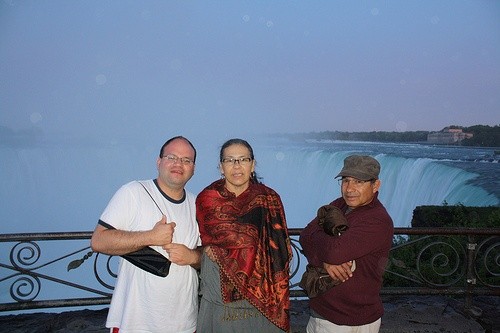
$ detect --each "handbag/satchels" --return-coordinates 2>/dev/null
[119,181,172,277]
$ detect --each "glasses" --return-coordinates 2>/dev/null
[223,158,252,164]
[163,154,194,164]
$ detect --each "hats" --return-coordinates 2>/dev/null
[334,155,381,180]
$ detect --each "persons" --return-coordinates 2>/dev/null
[195,138,294,333]
[299,155,394,333]
[90,136,202,333]
